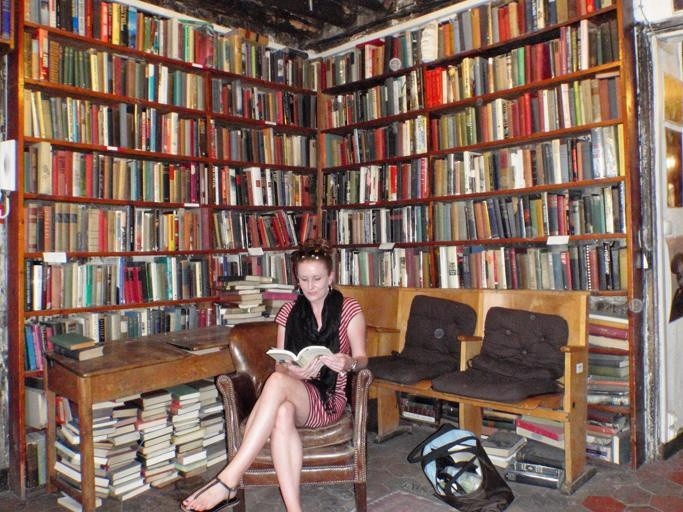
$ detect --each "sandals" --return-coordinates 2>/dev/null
[180,476,239,512]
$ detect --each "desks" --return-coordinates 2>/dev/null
[41,325,237,510]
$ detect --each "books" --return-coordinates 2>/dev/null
[267,345,333,368]
[24,377,226,510]
[1,0,215,370]
[421,2,629,469]
[398,395,459,430]
[322,29,432,287]
[208,26,319,327]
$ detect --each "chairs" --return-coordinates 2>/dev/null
[477,288,597,497]
[395,287,483,445]
[332,284,398,445]
[215,319,374,510]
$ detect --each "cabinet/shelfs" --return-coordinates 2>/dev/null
[651,27,682,462]
[318,3,645,473]
[0,2,318,510]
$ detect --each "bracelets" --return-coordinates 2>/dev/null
[351,359,358,372]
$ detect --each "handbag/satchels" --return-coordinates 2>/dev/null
[421,429,515,512]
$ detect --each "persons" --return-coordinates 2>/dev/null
[670,253,683,321]
[180,240,368,512]
